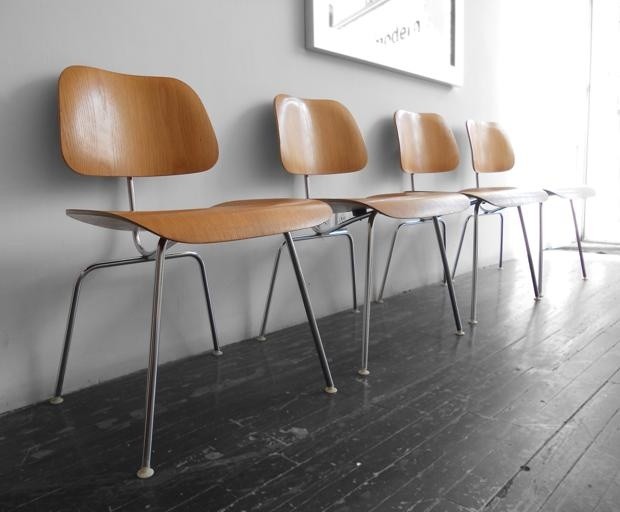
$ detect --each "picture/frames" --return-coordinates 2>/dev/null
[304,0,464,88]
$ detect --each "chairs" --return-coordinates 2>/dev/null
[48,65,337,478]
[255,95,470,376]
[452,121,597,297]
[374,110,548,325]
